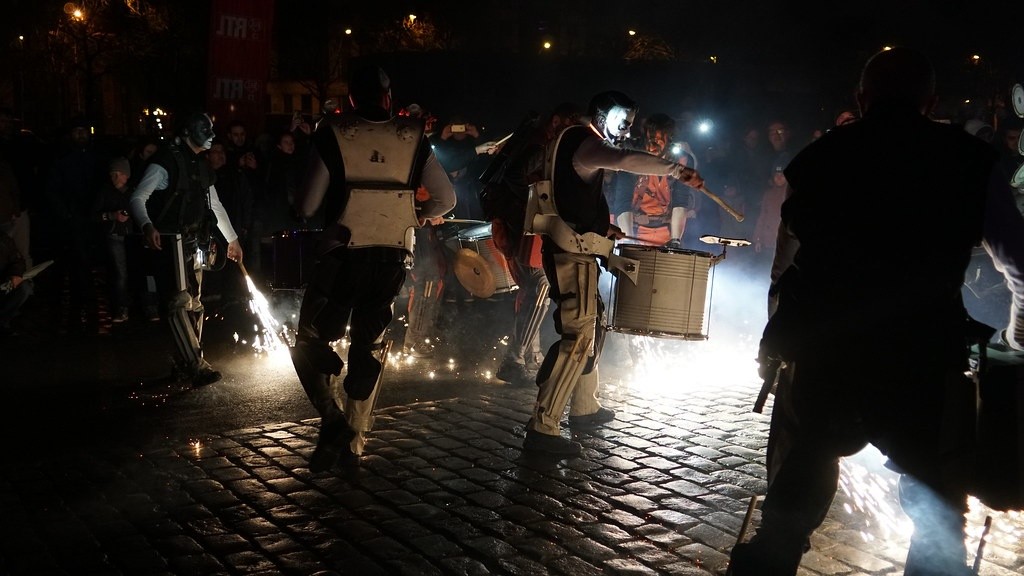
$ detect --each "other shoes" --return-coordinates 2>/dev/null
[522,431,582,454]
[568,408,613,424]
[191,368,222,388]
[309,414,356,474]
[149,316,160,321]
[339,450,360,470]
[111,307,129,322]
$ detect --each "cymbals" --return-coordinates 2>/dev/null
[452,249,495,298]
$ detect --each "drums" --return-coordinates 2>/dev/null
[607,239,715,344]
[964,329,1024,515]
[454,219,524,300]
[255,226,345,296]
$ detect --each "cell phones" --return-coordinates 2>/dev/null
[247,153,254,160]
[294,110,302,121]
[451,124,466,132]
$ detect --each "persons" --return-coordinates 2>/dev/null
[0,43,1024,357]
[293,61,458,479]
[491,103,580,386]
[523,91,705,456]
[130,112,242,388]
[726,48,1024,575]
